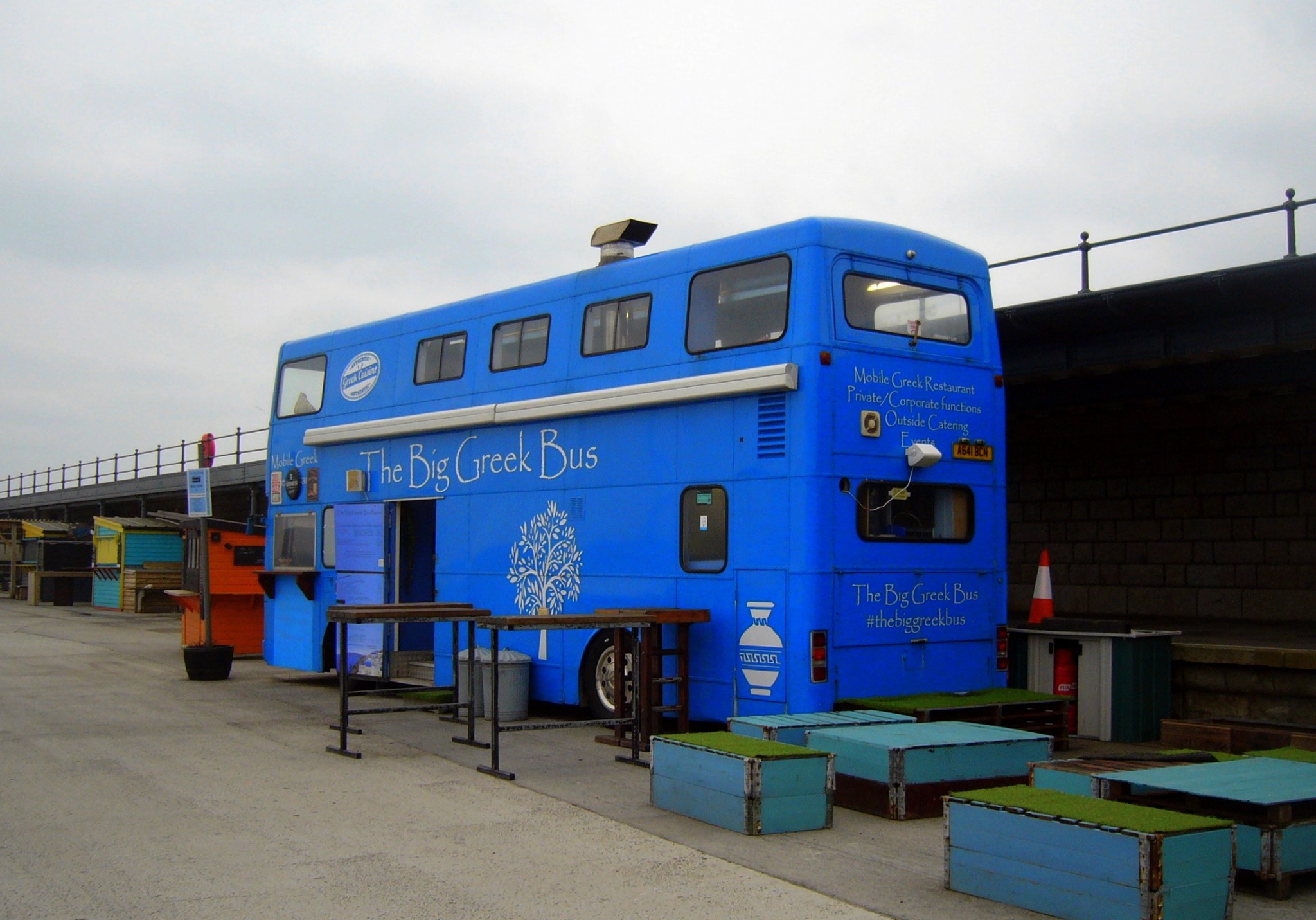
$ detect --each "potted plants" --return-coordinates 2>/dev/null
[185,518,231,679]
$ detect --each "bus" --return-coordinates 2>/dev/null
[246,219,1009,732]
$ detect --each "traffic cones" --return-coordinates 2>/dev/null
[1026,548,1055,623]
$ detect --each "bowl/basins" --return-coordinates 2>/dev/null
[278,558,292,566]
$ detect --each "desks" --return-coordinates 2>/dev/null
[806,719,1055,821]
[1093,755,1315,898]
[326,606,707,781]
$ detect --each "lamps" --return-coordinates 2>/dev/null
[907,443,941,468]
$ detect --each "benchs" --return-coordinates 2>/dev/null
[653,686,1316,920]
